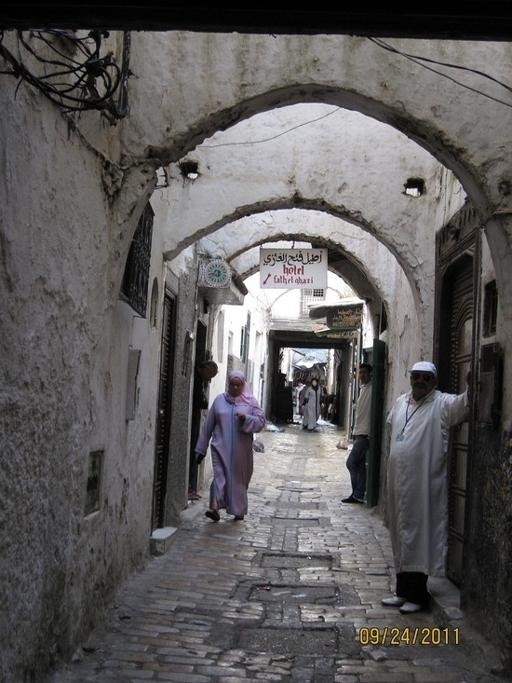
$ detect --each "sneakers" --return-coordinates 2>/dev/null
[205,510,220,521]
[340,494,364,503]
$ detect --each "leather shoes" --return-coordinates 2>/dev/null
[382,596,407,605]
[399,602,422,614]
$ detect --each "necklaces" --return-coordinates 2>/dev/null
[394,396,433,442]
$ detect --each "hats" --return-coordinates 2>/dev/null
[407,360,437,375]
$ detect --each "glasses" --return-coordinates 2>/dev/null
[410,372,430,381]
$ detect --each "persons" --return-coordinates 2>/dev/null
[380,360,473,614]
[187,361,218,499]
[193,369,265,522]
[292,377,326,431]
[340,362,375,503]
